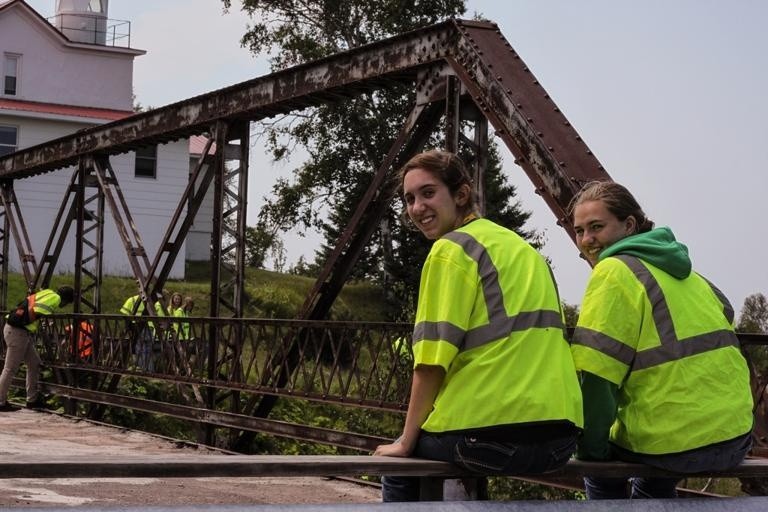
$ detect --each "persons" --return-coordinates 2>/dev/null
[0,285,74,412]
[568,182,755,500]
[122,290,195,374]
[63,310,94,362]
[373,152,584,502]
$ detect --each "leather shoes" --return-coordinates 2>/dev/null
[26,400,50,409]
[0,401,21,412]
